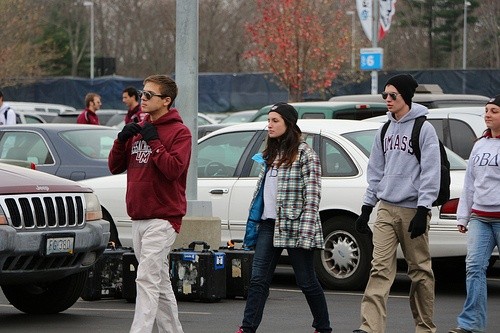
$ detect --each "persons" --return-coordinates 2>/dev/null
[108,75,191,333]
[352,73,441,333]
[236,102,333,333]
[448,96,500,333]
[76,92,102,125]
[122,87,146,126]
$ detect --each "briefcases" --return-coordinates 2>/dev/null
[169,241,227,303]
[122,252,139,304]
[217,239,256,298]
[79,242,133,301]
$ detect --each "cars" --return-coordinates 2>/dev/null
[0,93,493,181]
[71,118,500,292]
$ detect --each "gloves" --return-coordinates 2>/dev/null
[408,206,431,239]
[355,206,374,233]
[118,123,143,145]
[140,123,159,144]
[381,116,451,207]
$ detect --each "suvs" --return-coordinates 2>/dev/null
[0,160,111,312]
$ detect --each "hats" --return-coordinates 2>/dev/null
[268,102,298,123]
[384,74,418,106]
[486,95,500,107]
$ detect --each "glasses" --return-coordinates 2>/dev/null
[382,92,401,100]
[137,90,167,100]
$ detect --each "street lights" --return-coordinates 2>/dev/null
[84,1,95,80]
[347,10,355,71]
[462,0,470,93]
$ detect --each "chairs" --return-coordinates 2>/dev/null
[327,153,350,174]
[5,147,28,162]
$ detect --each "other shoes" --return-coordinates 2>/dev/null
[236,326,243,333]
[449,328,473,333]
[353,329,368,333]
